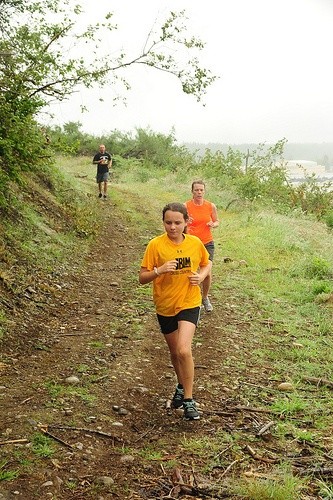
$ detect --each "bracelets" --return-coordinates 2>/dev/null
[154,267,160,275]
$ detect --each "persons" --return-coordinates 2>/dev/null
[58,137,61,142]
[183,180,220,312]
[46,137,51,143]
[93,144,112,198]
[139,203,210,421]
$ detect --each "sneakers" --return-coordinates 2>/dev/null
[182,400,200,421]
[171,383,184,409]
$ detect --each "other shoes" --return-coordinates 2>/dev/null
[202,297,214,312]
[102,195,107,201]
[98,194,103,200]
